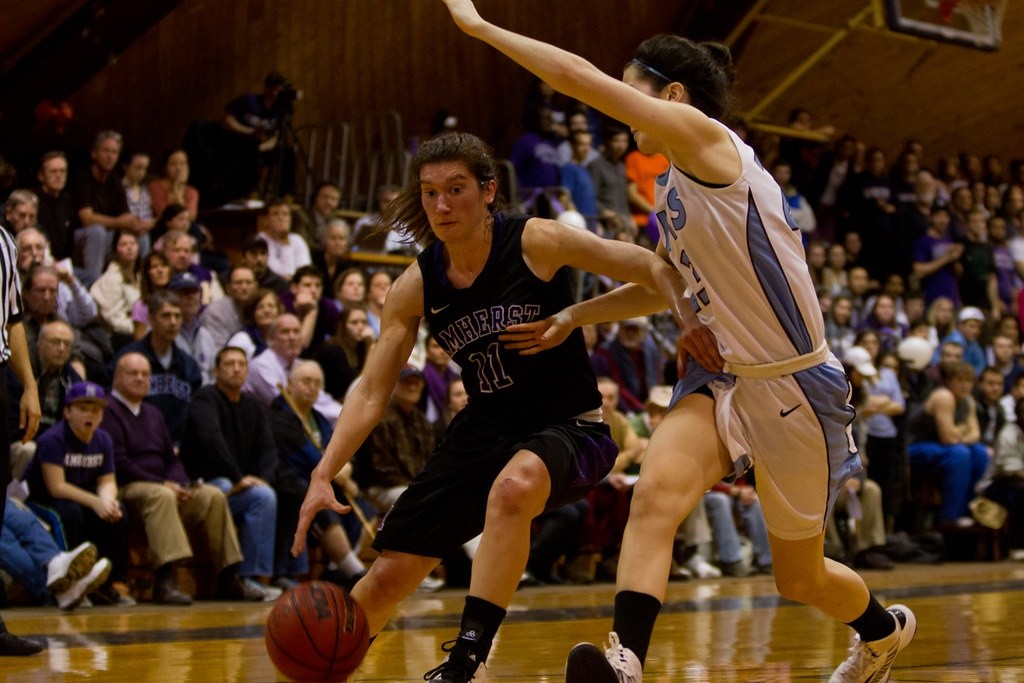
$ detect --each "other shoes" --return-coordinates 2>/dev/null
[218,578,265,602]
[419,576,443,591]
[685,557,720,578]
[855,550,894,572]
[725,561,754,578]
[153,585,194,607]
[320,563,363,587]
[108,591,136,608]
[669,558,692,580]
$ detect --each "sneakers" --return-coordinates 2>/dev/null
[565,629,644,683]
[46,541,97,593]
[422,639,485,683]
[828,602,916,683]
[56,558,113,611]
[1,632,43,656]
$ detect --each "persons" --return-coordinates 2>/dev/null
[0,223,44,654]
[443,0,917,683]
[727,108,1024,573]
[291,134,722,683]
[0,71,526,611]
[517,110,773,584]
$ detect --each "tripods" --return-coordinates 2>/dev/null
[254,107,319,203]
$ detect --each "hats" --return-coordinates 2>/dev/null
[168,274,200,294]
[646,384,671,409]
[843,344,878,378]
[66,382,110,407]
[399,367,424,383]
[958,308,986,322]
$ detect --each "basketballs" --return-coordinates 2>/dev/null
[264,580,371,682]
